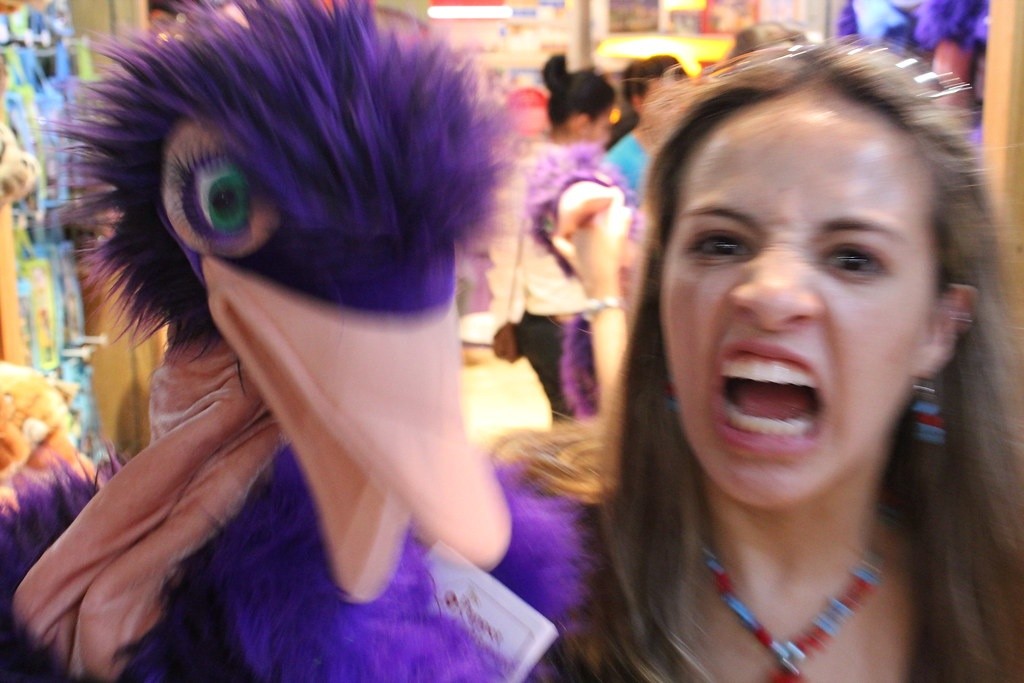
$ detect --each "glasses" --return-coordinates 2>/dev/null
[707,38,963,100]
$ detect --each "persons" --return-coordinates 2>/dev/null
[484,41,1024,683]
[507,54,689,426]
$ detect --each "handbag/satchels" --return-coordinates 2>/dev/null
[493,323,522,363]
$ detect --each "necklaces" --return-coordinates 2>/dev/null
[700,545,885,683]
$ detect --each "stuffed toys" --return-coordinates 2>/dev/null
[0,0,586,683]
